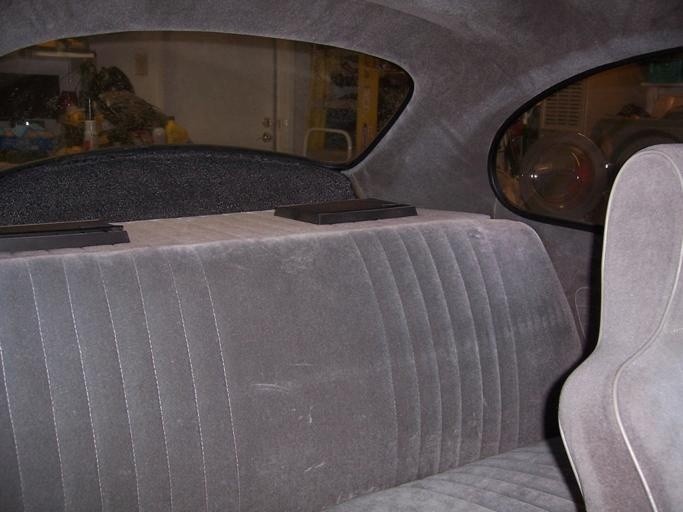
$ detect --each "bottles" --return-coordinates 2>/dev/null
[165,116,180,146]
[81,93,98,152]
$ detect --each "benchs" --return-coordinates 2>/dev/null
[0,218,584,512]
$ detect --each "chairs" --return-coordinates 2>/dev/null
[558,142,683,512]
